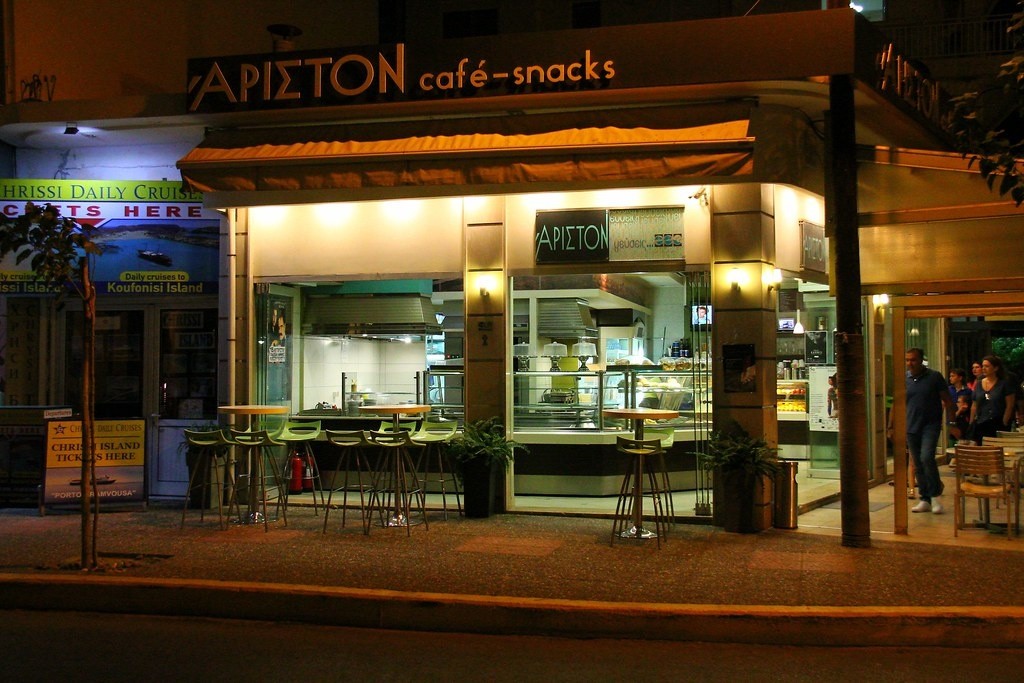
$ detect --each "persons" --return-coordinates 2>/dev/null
[888,347,1024,514]
[267,307,287,348]
[693,307,710,324]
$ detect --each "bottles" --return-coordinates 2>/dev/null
[683,339,691,358]
[679,338,685,357]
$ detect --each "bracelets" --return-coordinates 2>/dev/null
[950,422,956,425]
[888,427,893,430]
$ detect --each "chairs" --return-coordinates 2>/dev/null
[954,426,1024,538]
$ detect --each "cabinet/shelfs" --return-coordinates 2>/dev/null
[424,370,809,459]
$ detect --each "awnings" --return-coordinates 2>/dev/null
[176,100,814,194]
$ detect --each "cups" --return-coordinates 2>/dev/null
[783,367,792,380]
[791,368,798,379]
[799,366,806,379]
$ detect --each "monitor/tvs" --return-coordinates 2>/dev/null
[692,304,711,331]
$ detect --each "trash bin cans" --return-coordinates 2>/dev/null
[773,460,799,529]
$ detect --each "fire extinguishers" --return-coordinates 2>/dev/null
[301,446,314,493]
[287,449,303,494]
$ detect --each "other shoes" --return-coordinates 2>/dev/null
[912,500,931,512]
[908,489,915,499]
[931,497,943,514]
[950,457,957,467]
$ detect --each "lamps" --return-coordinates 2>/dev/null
[480,288,489,296]
[731,282,740,293]
[792,309,804,334]
[768,282,781,292]
[876,303,887,310]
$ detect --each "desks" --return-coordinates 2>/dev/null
[359,404,431,527]
[945,448,1024,520]
[218,405,291,525]
[602,408,679,540]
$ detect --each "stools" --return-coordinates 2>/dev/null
[367,430,429,537]
[408,421,462,520]
[611,436,666,550]
[626,426,675,532]
[276,421,325,518]
[367,421,421,515]
[180,430,241,531]
[322,429,385,534]
[226,430,288,531]
[231,420,285,516]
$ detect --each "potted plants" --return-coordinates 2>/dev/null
[684,419,786,533]
[443,414,530,517]
[176,421,234,510]
